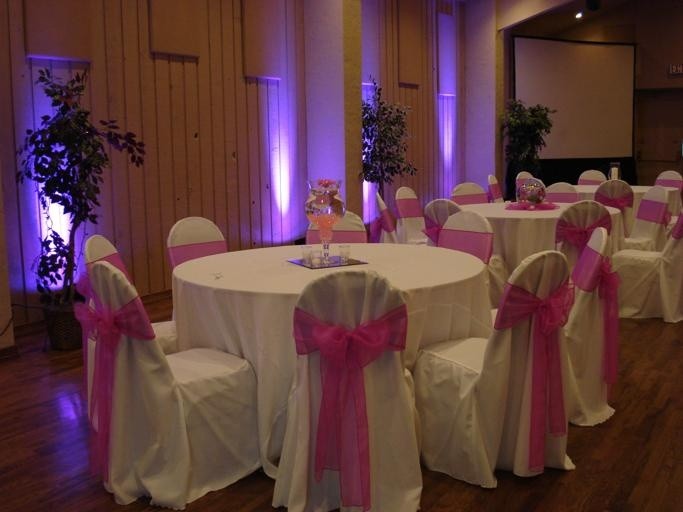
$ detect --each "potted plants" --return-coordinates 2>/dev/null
[11,66,146,349]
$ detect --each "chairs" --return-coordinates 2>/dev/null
[88,261,260,510]
[306,209,368,243]
[86,234,177,393]
[167,215,228,265]
[270,272,423,511]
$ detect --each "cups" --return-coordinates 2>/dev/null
[301,244,351,268]
